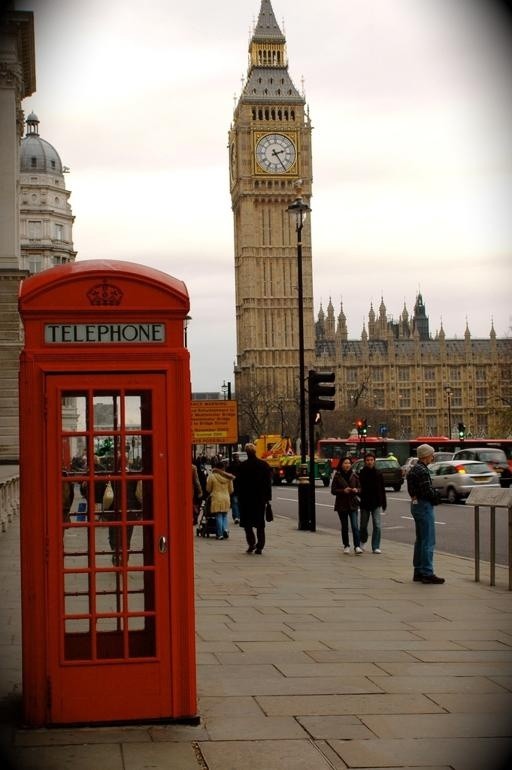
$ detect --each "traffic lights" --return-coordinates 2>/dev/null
[357,421,363,433]
[104,440,108,447]
[363,421,367,434]
[309,371,335,410]
[109,438,112,447]
[314,412,322,425]
[458,424,464,440]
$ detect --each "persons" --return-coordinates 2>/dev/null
[234,442,272,556]
[331,457,363,555]
[407,444,445,584]
[358,453,387,554]
[61,453,243,568]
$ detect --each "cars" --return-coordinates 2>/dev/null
[233,435,510,503]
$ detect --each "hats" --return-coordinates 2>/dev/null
[417,444,434,457]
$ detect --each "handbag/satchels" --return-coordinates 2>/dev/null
[266,504,273,521]
[103,482,114,510]
[77,496,87,521]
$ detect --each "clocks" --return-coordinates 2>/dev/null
[255,134,295,176]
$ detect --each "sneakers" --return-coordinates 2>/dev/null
[413,572,424,581]
[422,574,444,584]
[344,543,381,553]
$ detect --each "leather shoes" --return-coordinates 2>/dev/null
[246,545,256,552]
[255,544,263,554]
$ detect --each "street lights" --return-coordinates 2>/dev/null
[220,381,228,400]
[444,384,452,439]
[285,177,316,532]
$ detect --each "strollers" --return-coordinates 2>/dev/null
[195,495,227,541]
